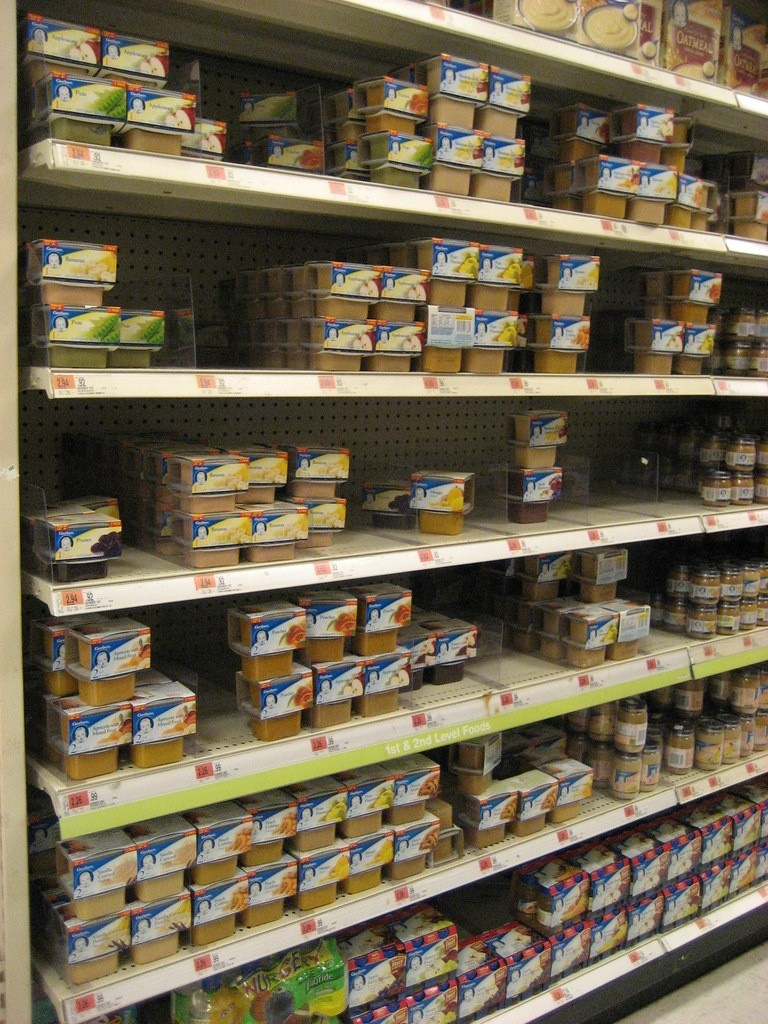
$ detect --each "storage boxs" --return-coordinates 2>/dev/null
[18,2,768,1024]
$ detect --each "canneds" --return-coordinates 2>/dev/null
[702,306,768,376]
[564,659,768,800]
[634,421,768,508]
[649,555,768,640]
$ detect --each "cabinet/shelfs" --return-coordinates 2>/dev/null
[598,1,768,972]
[0,1,738,1024]
